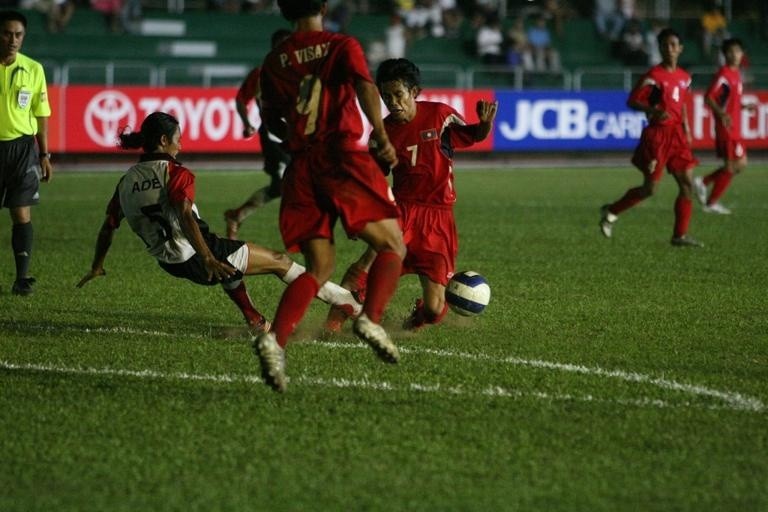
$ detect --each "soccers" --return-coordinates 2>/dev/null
[442,268,492,319]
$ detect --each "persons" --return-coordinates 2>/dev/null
[623,20,649,69]
[506,19,535,72]
[323,58,499,351]
[224,30,296,240]
[479,53,500,67]
[75,112,365,343]
[89,1,133,36]
[692,37,748,215]
[598,29,704,248]
[475,12,503,64]
[45,0,75,33]
[0,12,53,296]
[165,1,186,19]
[703,6,734,65]
[251,0,407,393]
[525,15,562,72]
[495,35,522,66]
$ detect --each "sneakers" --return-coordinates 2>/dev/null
[352,314,400,363]
[403,313,424,331]
[253,333,286,394]
[694,176,708,205]
[12,276,36,297]
[701,201,730,215]
[347,288,367,318]
[671,237,704,247]
[598,204,618,238]
[224,209,238,240]
